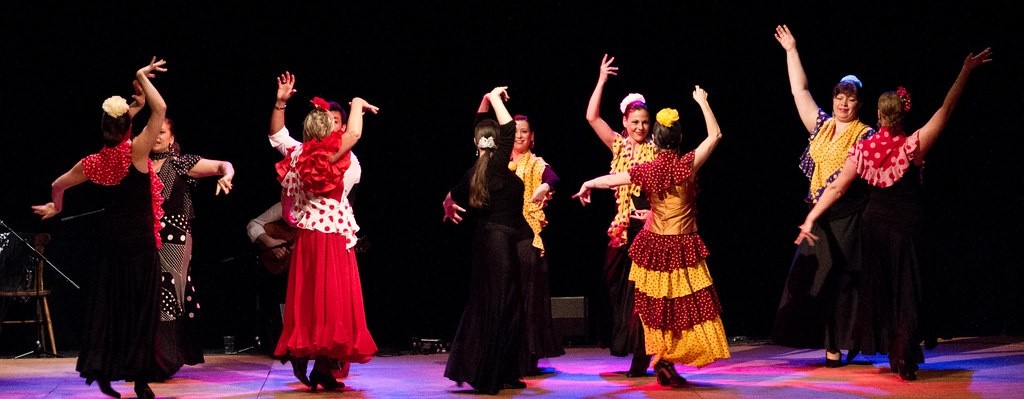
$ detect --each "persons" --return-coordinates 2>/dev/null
[792,42,995,382]
[438,82,561,396]
[570,84,730,387]
[245,202,302,353]
[266,68,382,392]
[583,53,668,379]
[31,55,169,399]
[121,77,236,383]
[773,21,875,366]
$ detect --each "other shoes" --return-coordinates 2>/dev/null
[527,369,543,375]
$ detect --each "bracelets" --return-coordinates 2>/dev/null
[274,103,286,110]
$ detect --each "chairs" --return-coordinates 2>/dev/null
[0,233,58,359]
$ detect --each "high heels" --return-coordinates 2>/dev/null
[890,355,915,379]
[86,377,120,399]
[839,349,849,364]
[627,351,651,376]
[825,350,841,367]
[505,378,525,388]
[457,380,500,396]
[310,369,345,391]
[281,350,312,386]
[654,360,686,385]
[135,385,154,399]
